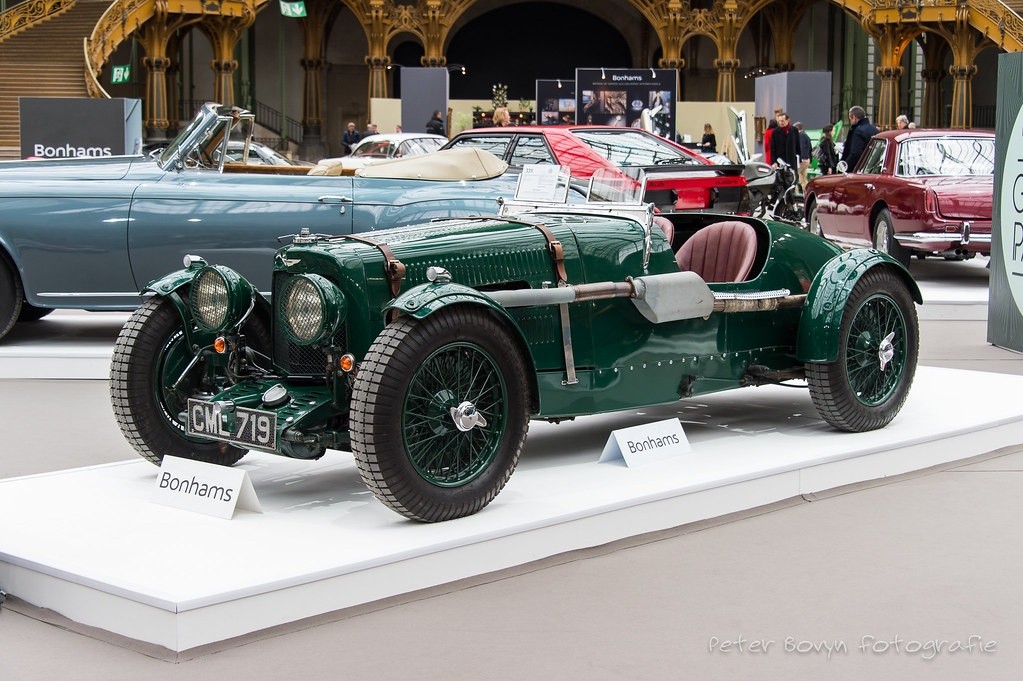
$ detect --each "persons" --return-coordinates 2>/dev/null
[792,121,814,192]
[815,124,841,173]
[837,104,879,171]
[763,119,775,164]
[340,91,722,158]
[896,115,909,130]
[768,108,784,129]
[768,114,796,173]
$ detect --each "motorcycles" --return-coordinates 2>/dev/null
[726,157,807,232]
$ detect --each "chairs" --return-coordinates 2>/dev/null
[674,220,757,281]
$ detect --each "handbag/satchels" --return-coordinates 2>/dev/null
[811,145,828,168]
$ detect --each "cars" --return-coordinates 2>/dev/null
[106,161,928,524]
[316,128,448,168]
[439,124,749,224]
[802,124,997,270]
[0,102,670,342]
[216,139,304,175]
